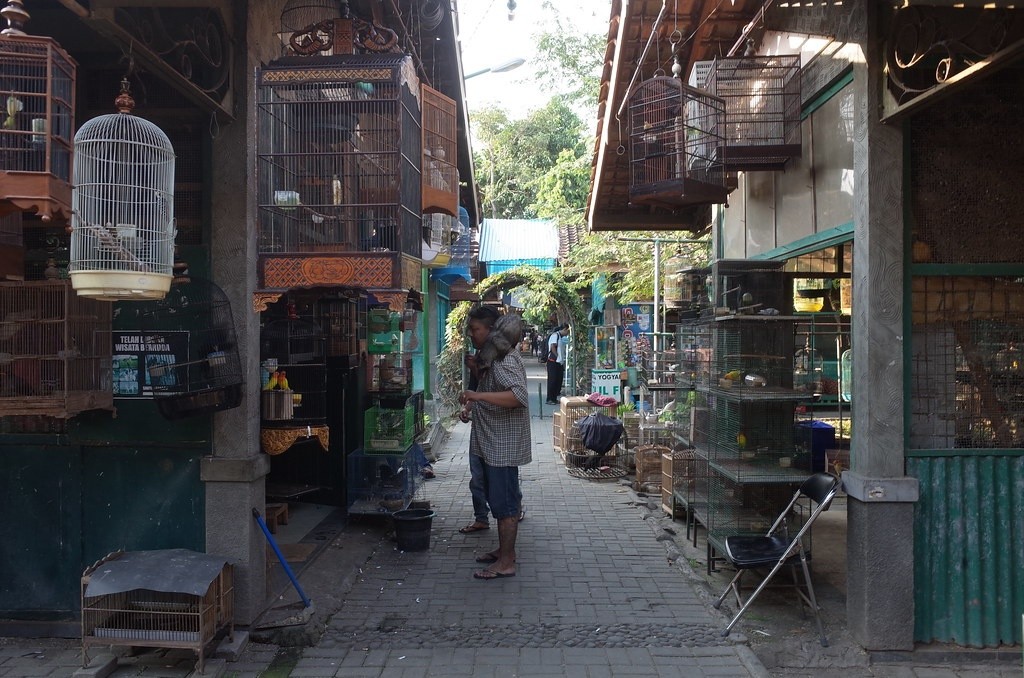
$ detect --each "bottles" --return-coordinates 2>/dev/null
[744,373,767,388]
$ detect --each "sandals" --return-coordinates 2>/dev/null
[458,523,490,533]
[554,400,560,404]
[515,505,526,521]
[476,553,516,563]
[545,401,556,405]
[474,563,515,578]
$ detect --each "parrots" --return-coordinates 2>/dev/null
[736,430,746,447]
[278,370,290,391]
[724,370,741,380]
[262,372,280,391]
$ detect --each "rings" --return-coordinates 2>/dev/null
[462,395,466,398]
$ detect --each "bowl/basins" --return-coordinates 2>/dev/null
[274,191,299,210]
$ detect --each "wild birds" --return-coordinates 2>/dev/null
[156,189,167,217]
[758,308,780,316]
[659,399,677,415]
[331,174,343,203]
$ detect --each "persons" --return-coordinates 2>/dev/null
[458,308,525,533]
[529,323,571,407]
[458,304,533,578]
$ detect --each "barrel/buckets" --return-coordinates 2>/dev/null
[392,509,436,552]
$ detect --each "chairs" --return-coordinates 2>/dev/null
[712,472,845,649]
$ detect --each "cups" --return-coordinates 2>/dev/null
[116,224,137,240]
[779,458,791,468]
[31,118,47,142]
[208,351,226,367]
[720,379,732,389]
[261,390,293,420]
